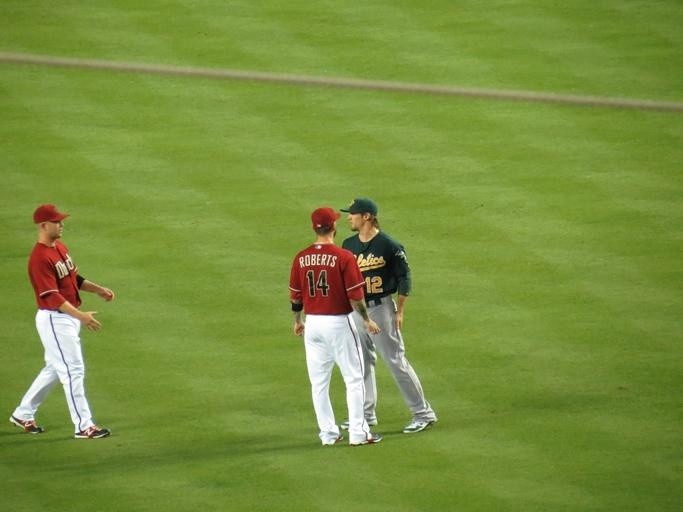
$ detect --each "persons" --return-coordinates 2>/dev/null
[7,203,116,440]
[288,208,383,446]
[340,196,438,435]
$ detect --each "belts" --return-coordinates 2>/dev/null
[366,298,382,308]
[40,307,63,313]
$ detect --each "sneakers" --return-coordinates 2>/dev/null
[403,414,438,434]
[322,431,344,446]
[10,415,44,434]
[341,415,378,430]
[75,425,111,439]
[350,432,382,445]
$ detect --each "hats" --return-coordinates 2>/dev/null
[33,204,70,224]
[340,198,378,216]
[311,208,341,228]
